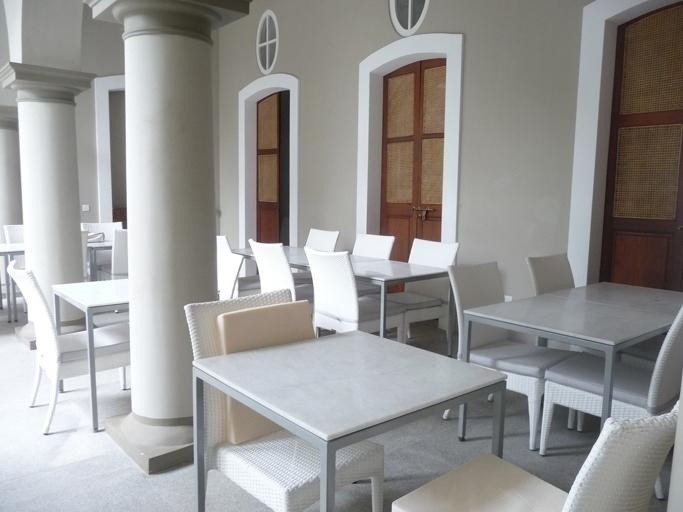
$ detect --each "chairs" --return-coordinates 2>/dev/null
[349,233,392,260]
[0,220,244,435]
[383,236,456,357]
[247,239,296,300]
[303,226,340,257]
[389,399,680,510]
[210,296,389,510]
[302,247,406,346]
[442,255,578,446]
[519,252,613,359]
[536,303,680,499]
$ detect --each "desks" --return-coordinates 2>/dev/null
[454,278,680,451]
[189,327,511,512]
